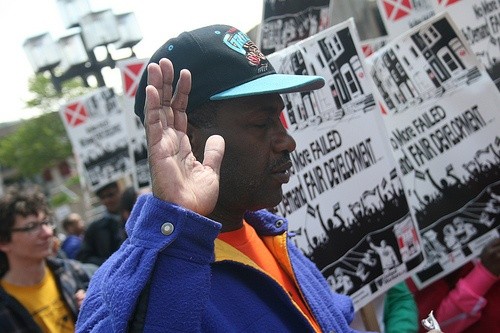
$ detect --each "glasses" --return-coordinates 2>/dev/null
[6,215,56,238]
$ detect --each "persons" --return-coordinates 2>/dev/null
[0,182,140,333]
[73,24,357,333]
[381,236,500,333]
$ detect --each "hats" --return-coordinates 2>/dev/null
[134,24,325,125]
